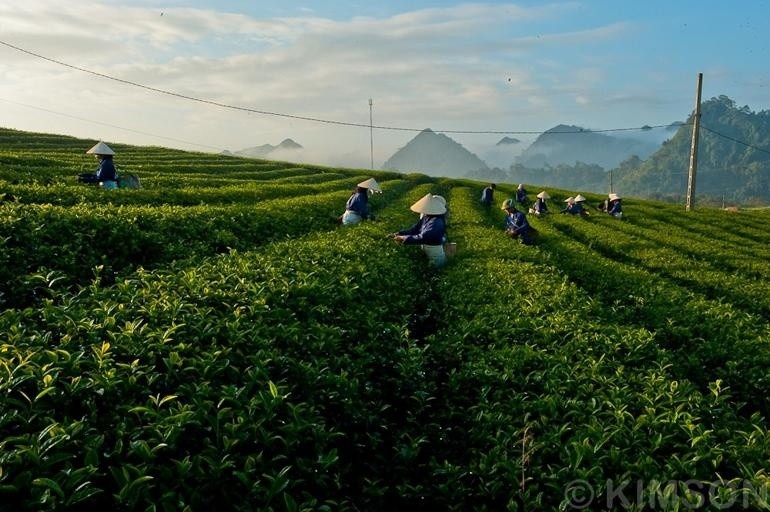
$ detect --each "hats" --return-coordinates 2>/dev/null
[537,190,551,199]
[564,197,575,203]
[358,178,382,193]
[501,199,514,211]
[410,192,448,215]
[86,141,117,155]
[575,194,586,201]
[609,194,623,203]
[433,194,447,207]
[518,183,526,191]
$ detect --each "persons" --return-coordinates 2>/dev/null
[560,194,622,220]
[387,193,447,266]
[516,184,526,204]
[481,184,496,205]
[342,178,383,226]
[78,142,120,187]
[533,191,551,214]
[501,199,531,244]
[419,195,447,237]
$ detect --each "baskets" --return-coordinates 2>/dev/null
[117,174,142,191]
[529,207,536,214]
[444,241,458,259]
